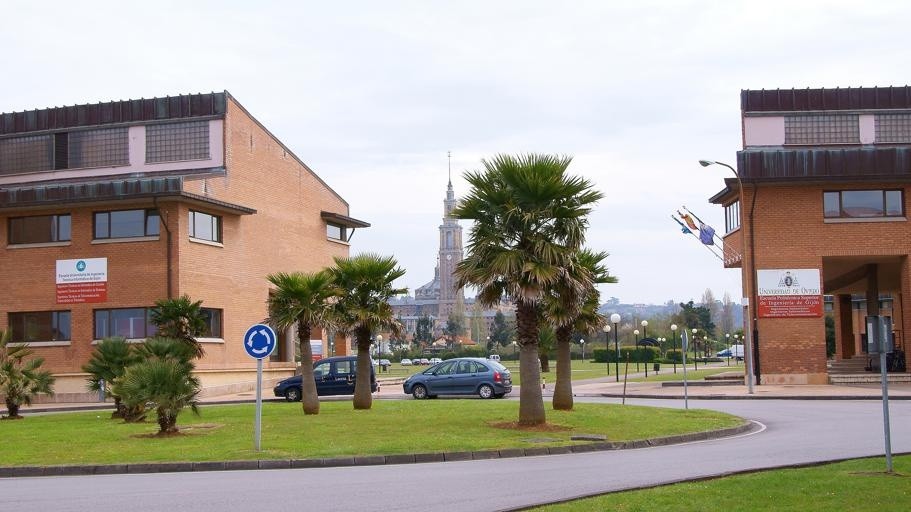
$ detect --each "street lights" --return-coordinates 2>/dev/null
[459,340,463,357]
[330,342,334,357]
[733,333,739,366]
[579,339,585,363]
[742,336,745,344]
[486,335,491,358]
[699,160,754,394]
[432,342,437,366]
[511,339,517,365]
[377,335,383,373]
[602,313,709,382]
[726,334,730,366]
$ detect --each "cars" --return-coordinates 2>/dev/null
[717,349,732,357]
[404,357,513,400]
[373,358,444,367]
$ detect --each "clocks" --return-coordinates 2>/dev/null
[447,254,453,261]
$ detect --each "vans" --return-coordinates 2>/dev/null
[274,355,383,402]
[731,344,745,361]
[489,355,500,362]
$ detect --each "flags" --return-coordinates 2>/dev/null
[699,224,715,245]
[680,224,691,234]
[680,214,696,231]
